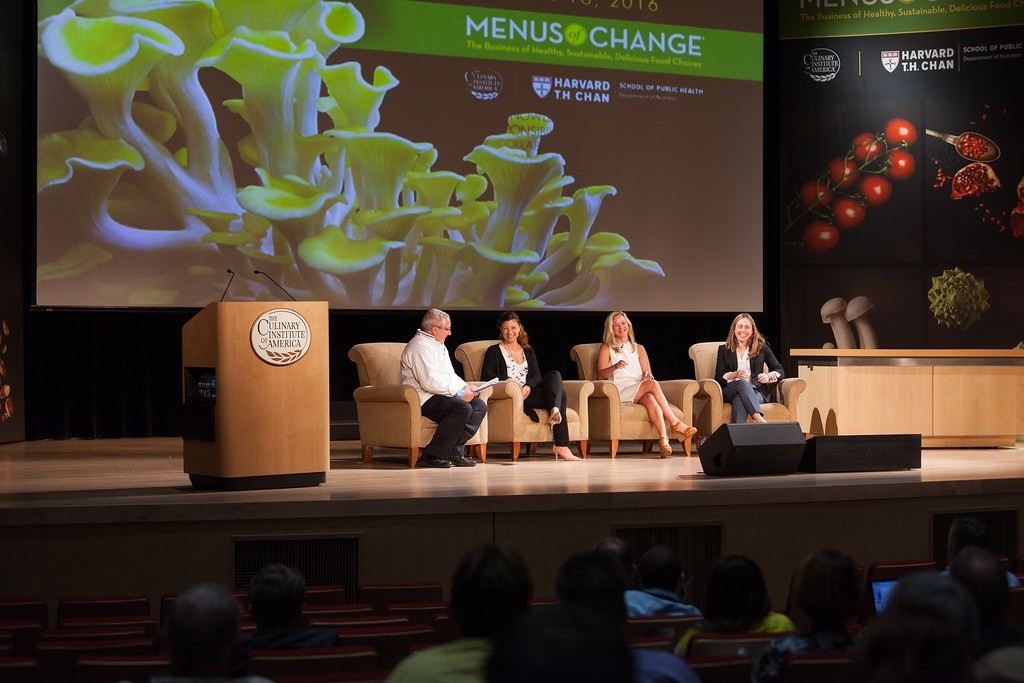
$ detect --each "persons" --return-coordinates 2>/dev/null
[151,517,1024,683]
[400,308,488,468]
[715,313,785,424]
[481,311,582,461]
[596,311,698,457]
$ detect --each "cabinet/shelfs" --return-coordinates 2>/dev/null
[787,348,1024,452]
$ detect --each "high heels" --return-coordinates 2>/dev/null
[659,436,672,458]
[552,444,582,461]
[669,421,698,443]
[547,412,562,430]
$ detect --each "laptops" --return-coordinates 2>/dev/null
[871,577,899,612]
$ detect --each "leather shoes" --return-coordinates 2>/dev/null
[417,451,455,467]
[446,455,477,467]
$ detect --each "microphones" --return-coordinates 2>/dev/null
[220,269,235,302]
[253,270,296,302]
[621,345,624,348]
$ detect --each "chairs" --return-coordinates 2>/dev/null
[0,537,1024,683]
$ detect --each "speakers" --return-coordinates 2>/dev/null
[697,420,806,477]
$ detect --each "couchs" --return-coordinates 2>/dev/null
[685,338,807,444]
[564,341,698,459]
[347,341,493,464]
[454,340,597,462]
